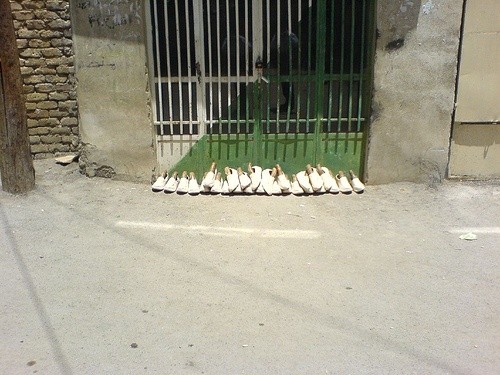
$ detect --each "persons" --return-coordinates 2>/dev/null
[220,20,256,123]
[266,20,302,112]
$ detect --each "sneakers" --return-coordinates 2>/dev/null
[152,162,366,195]
